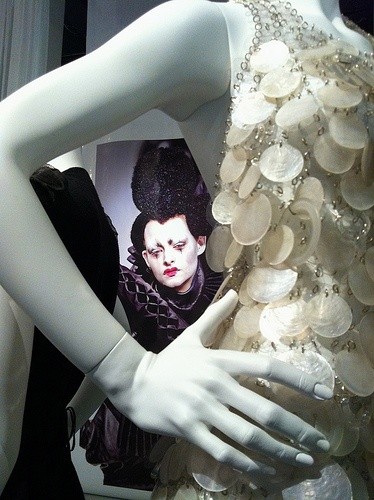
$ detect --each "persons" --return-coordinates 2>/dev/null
[1,0,373,498]
[102,142,225,490]
[0,150,117,499]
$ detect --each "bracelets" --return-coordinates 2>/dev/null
[64,405,75,451]
